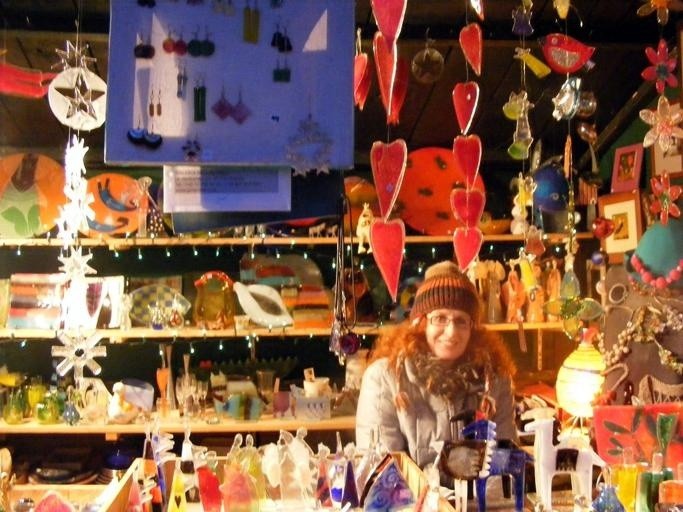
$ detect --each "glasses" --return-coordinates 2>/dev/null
[426,316,475,329]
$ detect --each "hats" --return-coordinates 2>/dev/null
[411,261,480,320]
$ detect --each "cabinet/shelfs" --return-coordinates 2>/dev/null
[2,232,596,511]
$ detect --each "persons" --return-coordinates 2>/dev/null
[353,259,515,499]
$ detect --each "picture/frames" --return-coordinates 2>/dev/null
[597,98,683,266]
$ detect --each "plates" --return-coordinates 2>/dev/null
[396,146,488,236]
[1,152,70,240]
[75,173,150,240]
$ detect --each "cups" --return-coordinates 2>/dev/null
[273,392,290,416]
[303,377,332,398]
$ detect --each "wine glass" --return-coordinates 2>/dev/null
[175,374,209,418]
